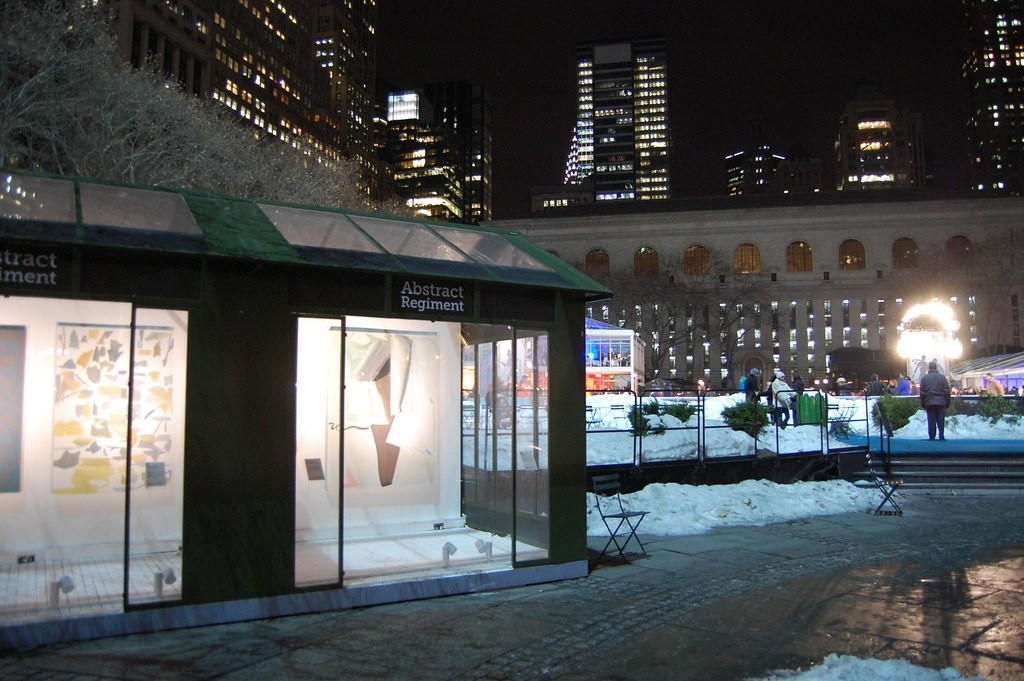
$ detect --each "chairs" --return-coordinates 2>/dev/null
[611,404,629,428]
[827,406,859,439]
[592,473,650,565]
[586,406,602,429]
[865,454,904,515]
[462,404,548,434]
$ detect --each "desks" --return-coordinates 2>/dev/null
[596,406,611,429]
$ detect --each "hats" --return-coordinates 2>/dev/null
[750,368,759,374]
[835,377,846,383]
[871,374,879,380]
[777,372,785,378]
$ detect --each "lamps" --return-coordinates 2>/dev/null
[443,541,457,567]
[475,539,494,562]
[49,574,76,609]
[153,566,177,596]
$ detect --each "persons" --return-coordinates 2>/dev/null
[827,372,913,396]
[920,362,950,441]
[744,368,804,430]
[912,357,944,387]
[951,383,1024,399]
[985,373,1004,397]
